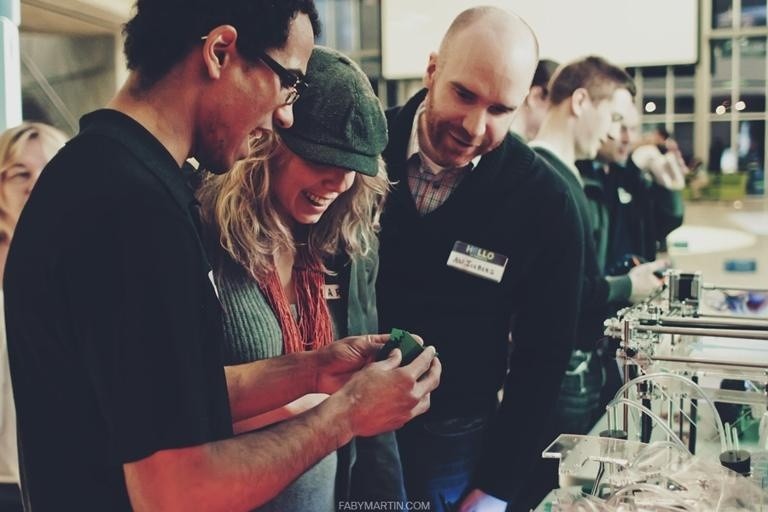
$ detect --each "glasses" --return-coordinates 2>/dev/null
[200,35,308,106]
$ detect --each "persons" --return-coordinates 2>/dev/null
[0,1,442,512]
[576,97,658,314]
[194,46,401,512]
[510,61,561,144]
[1,119,74,511]
[379,4,589,512]
[519,57,674,448]
[657,131,685,216]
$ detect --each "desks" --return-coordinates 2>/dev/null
[670,198,768,292]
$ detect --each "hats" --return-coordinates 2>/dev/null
[272,45,389,177]
